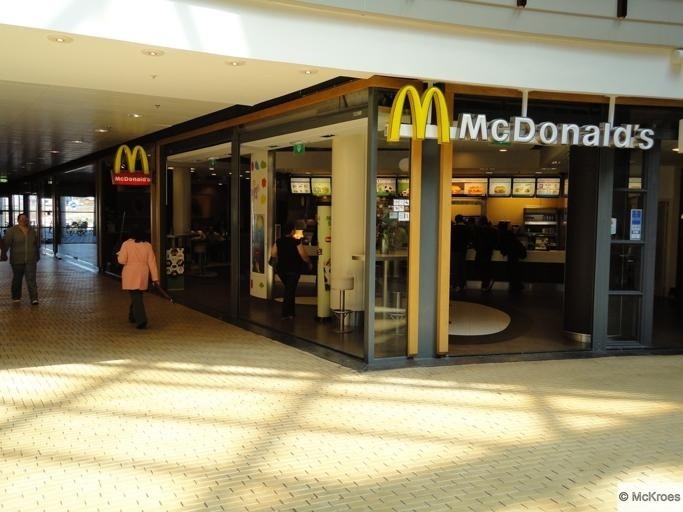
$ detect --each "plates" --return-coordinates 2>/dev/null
[493,183,509,195]
[512,183,532,195]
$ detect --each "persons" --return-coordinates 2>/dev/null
[0,213,40,306]
[115,224,160,328]
[548,210,566,252]
[269,214,310,318]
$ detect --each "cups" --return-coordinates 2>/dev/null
[401,187,408,196]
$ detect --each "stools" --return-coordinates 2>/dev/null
[329,274,354,334]
